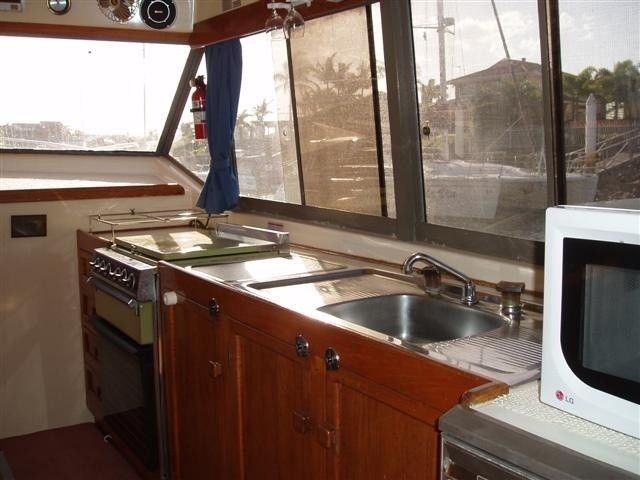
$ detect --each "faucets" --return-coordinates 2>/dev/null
[403,252,478,305]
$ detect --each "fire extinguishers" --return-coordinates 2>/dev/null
[189,75,208,139]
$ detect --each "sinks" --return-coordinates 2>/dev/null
[318,293,511,341]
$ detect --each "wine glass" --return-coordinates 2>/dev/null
[261,1,308,40]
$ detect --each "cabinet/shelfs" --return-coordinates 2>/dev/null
[156,260,509,480]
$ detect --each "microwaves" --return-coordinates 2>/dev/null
[539,203,640,442]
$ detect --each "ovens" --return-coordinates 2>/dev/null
[86,247,159,474]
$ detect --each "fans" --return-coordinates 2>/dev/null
[96,0,144,23]
